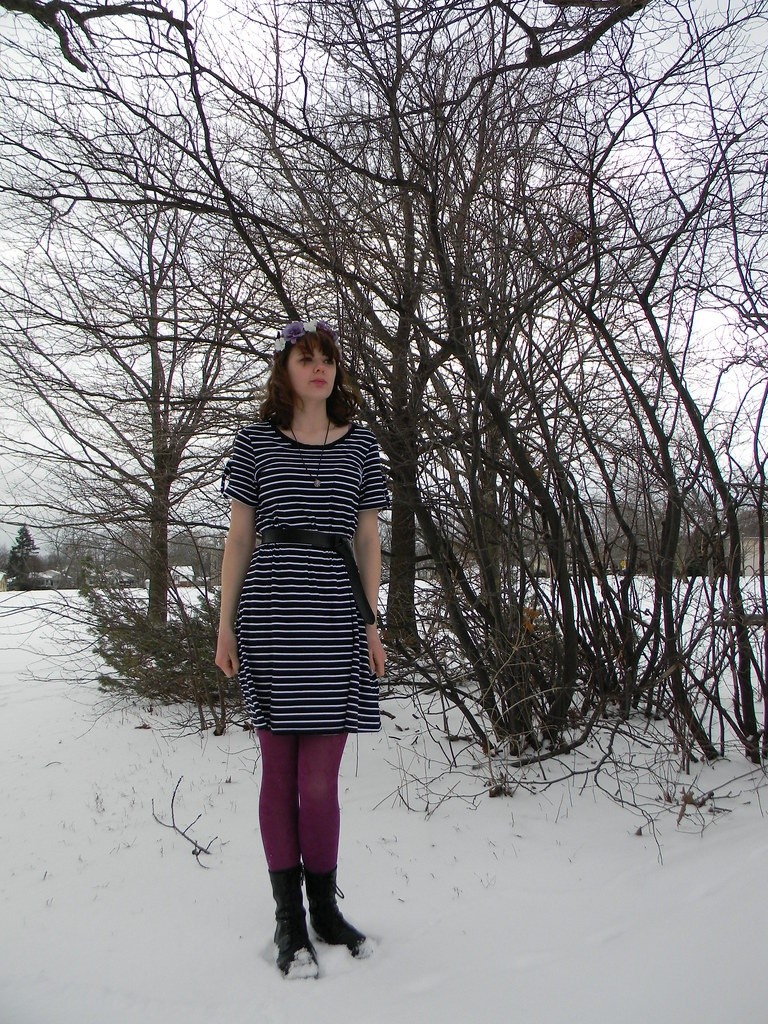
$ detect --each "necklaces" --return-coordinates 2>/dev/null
[290,420,331,488]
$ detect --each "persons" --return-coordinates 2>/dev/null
[214,322,388,980]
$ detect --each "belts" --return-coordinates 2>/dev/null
[261,529,376,625]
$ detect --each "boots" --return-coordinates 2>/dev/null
[267,863,319,978]
[304,865,367,956]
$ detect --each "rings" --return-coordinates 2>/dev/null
[384,659,388,662]
